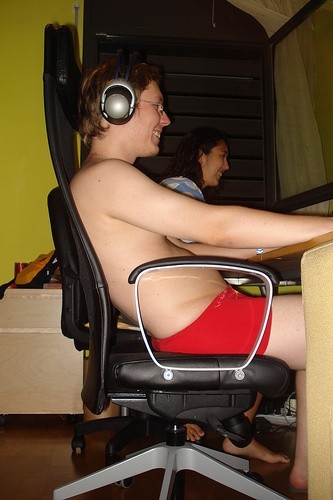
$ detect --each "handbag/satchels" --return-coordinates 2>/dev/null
[0,249,60,299]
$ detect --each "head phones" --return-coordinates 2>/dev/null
[99,49,137,125]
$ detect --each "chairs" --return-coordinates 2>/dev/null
[42,22,295,500]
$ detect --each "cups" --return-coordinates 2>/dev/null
[15,262,28,278]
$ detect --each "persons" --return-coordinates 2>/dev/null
[70,54,333,490]
[161,127,289,464]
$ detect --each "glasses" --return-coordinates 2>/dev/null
[139,99,163,116]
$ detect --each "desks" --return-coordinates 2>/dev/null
[249,231,333,500]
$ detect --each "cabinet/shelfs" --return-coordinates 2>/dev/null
[0,288,90,415]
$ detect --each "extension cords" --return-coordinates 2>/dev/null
[257,414,297,427]
